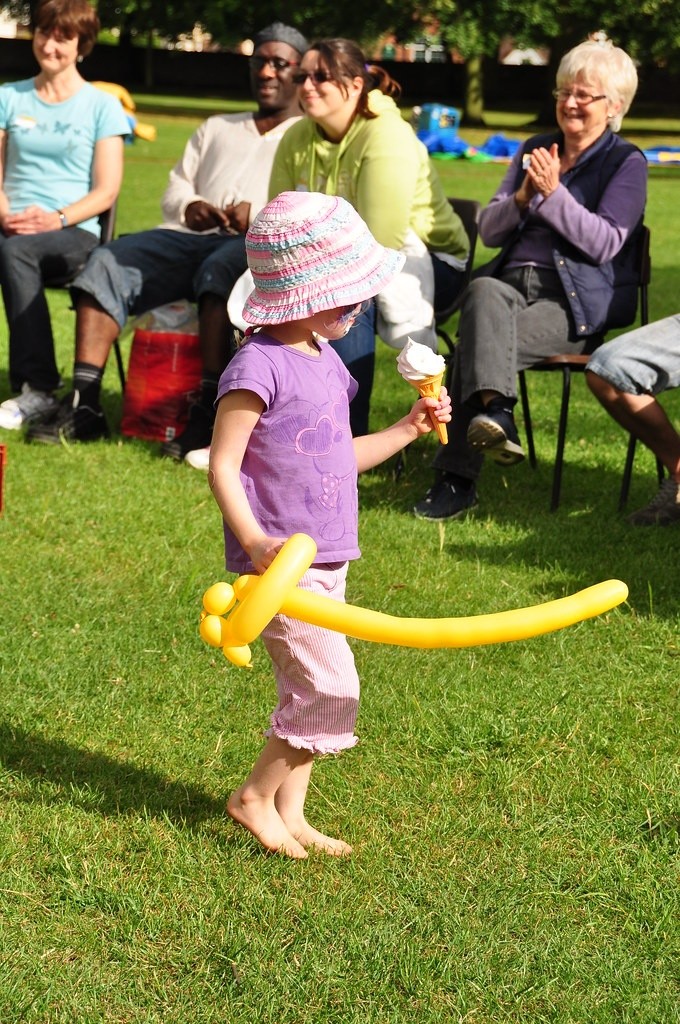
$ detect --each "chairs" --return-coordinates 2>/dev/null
[44,81,664,514]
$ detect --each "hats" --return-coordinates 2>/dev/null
[243,191,405,325]
[254,23,308,56]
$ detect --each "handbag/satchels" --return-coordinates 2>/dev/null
[121,329,202,442]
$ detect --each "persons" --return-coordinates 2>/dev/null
[413,29,648,523]
[583,313,680,526]
[24,21,309,462]
[206,189,453,858]
[0,0,134,430]
[183,38,472,478]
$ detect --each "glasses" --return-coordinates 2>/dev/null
[250,56,300,70]
[552,87,607,104]
[292,71,335,84]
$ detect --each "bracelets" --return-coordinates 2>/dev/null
[57,210,67,228]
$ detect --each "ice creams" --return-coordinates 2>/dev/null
[396,336,448,445]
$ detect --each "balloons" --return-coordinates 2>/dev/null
[200,533,629,668]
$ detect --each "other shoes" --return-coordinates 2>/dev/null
[0,383,56,429]
[184,445,210,470]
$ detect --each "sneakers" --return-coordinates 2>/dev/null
[160,381,217,458]
[466,408,525,466]
[623,480,680,526]
[413,473,479,521]
[28,391,108,443]
